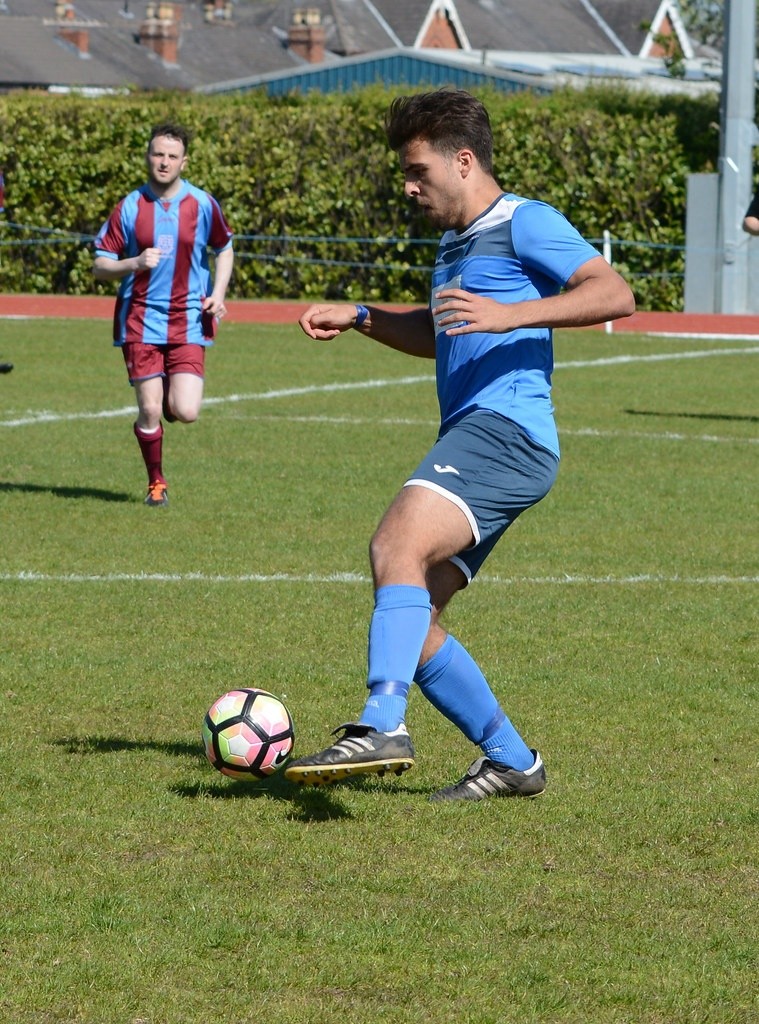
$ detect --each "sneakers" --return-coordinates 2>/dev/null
[427,749,547,804]
[285,721,416,781]
[144,479,167,504]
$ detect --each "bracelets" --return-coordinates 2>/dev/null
[355,304,368,324]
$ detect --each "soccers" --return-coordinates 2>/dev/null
[202,687,298,782]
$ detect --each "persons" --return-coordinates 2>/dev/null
[743,183,759,235]
[286,87,637,801]
[91,124,235,503]
